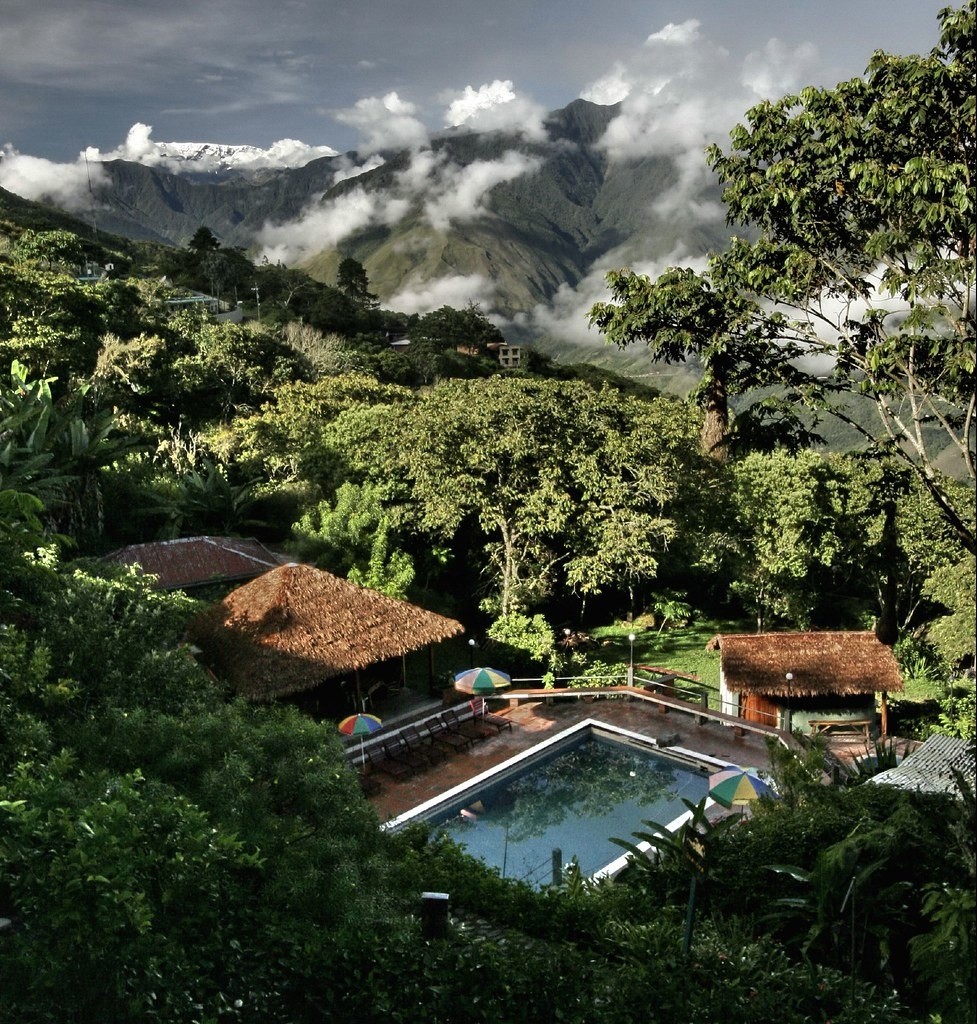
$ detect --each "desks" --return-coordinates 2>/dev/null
[808,719,872,752]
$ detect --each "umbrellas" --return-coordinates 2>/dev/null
[455,668,510,719]
[338,714,385,771]
[708,765,780,824]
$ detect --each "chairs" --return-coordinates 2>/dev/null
[400,726,447,766]
[364,743,413,783]
[424,717,470,752]
[441,710,486,747]
[470,697,513,734]
[348,757,381,799]
[382,735,428,776]
